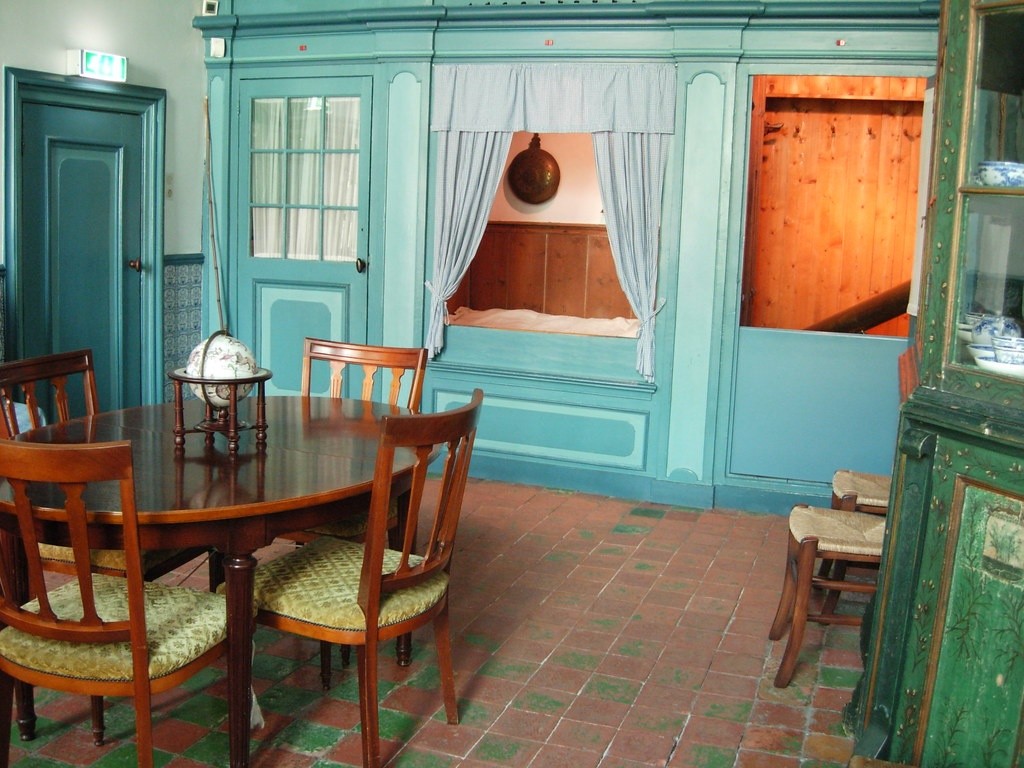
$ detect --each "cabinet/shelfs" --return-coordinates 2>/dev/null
[850,0,1024,768]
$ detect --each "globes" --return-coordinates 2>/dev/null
[185,330,258,432]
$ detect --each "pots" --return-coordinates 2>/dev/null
[508,133,560,205]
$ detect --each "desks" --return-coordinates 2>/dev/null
[2,395,440,768]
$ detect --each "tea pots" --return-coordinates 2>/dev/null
[972,310,1022,344]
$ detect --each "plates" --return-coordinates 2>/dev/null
[967,345,995,357]
[974,357,1023,382]
[959,324,973,342]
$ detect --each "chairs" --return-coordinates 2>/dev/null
[217,388,482,768]
[0,438,265,768]
[0,349,227,593]
[769,469,892,689]
[278,337,429,667]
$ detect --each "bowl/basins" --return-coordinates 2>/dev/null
[971,162,1024,189]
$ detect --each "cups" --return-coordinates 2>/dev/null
[964,312,982,324]
[991,344,1024,364]
[991,336,1024,347]
